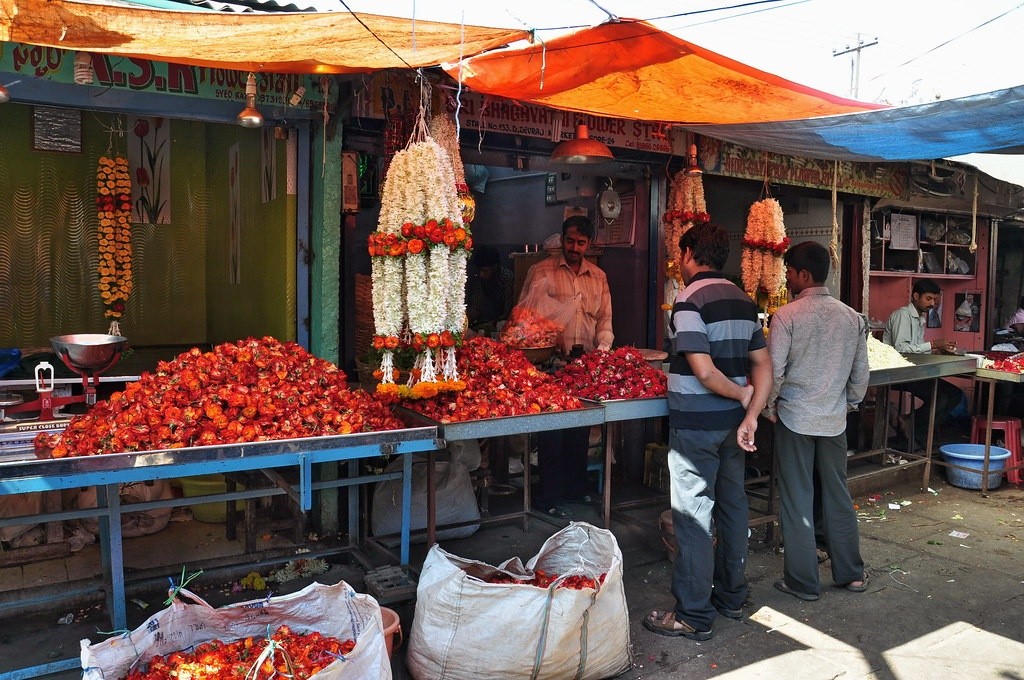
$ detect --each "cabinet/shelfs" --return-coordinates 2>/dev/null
[869,208,977,278]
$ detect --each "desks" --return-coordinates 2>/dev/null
[0,345,1024,680]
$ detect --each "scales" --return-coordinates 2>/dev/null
[518,344,557,372]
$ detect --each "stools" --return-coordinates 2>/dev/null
[971,414,1024,484]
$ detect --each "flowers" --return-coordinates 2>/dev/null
[467,564,607,591]
[122,625,355,680]
[34,114,790,460]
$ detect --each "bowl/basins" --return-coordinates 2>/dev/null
[939,443,1012,490]
[1009,323,1024,337]
[177,476,247,523]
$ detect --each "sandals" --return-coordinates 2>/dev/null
[643,610,712,640]
[847,569,870,592]
[711,594,744,619]
[538,501,574,520]
[567,491,603,506]
[774,578,821,601]
[816,547,828,561]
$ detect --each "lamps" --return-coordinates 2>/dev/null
[237,72,263,126]
[550,117,615,165]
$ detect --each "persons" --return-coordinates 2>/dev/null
[517,214,615,521]
[994,300,1024,449]
[765,242,872,602]
[639,224,774,639]
[883,278,970,457]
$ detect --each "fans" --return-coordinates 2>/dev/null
[909,160,957,198]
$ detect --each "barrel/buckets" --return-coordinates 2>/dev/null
[380,606,402,661]
[659,509,717,562]
